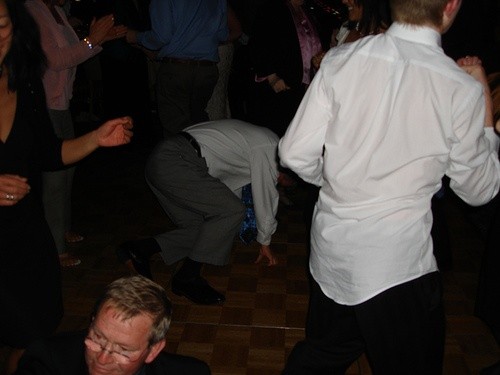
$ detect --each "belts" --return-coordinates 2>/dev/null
[177,131,202,158]
[160,57,213,66]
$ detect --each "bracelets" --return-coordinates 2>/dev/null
[84,38,93,52]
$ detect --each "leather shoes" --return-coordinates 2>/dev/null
[172,272,225,307]
[117,239,154,282]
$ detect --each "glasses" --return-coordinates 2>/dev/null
[83,336,141,362]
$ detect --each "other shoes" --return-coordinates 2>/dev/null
[479,360,500,375]
[63,233,84,242]
[59,253,82,267]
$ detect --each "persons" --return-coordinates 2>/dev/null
[279,0,500,374]
[0,0,500,375]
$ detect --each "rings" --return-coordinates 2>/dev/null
[5,192,15,200]
